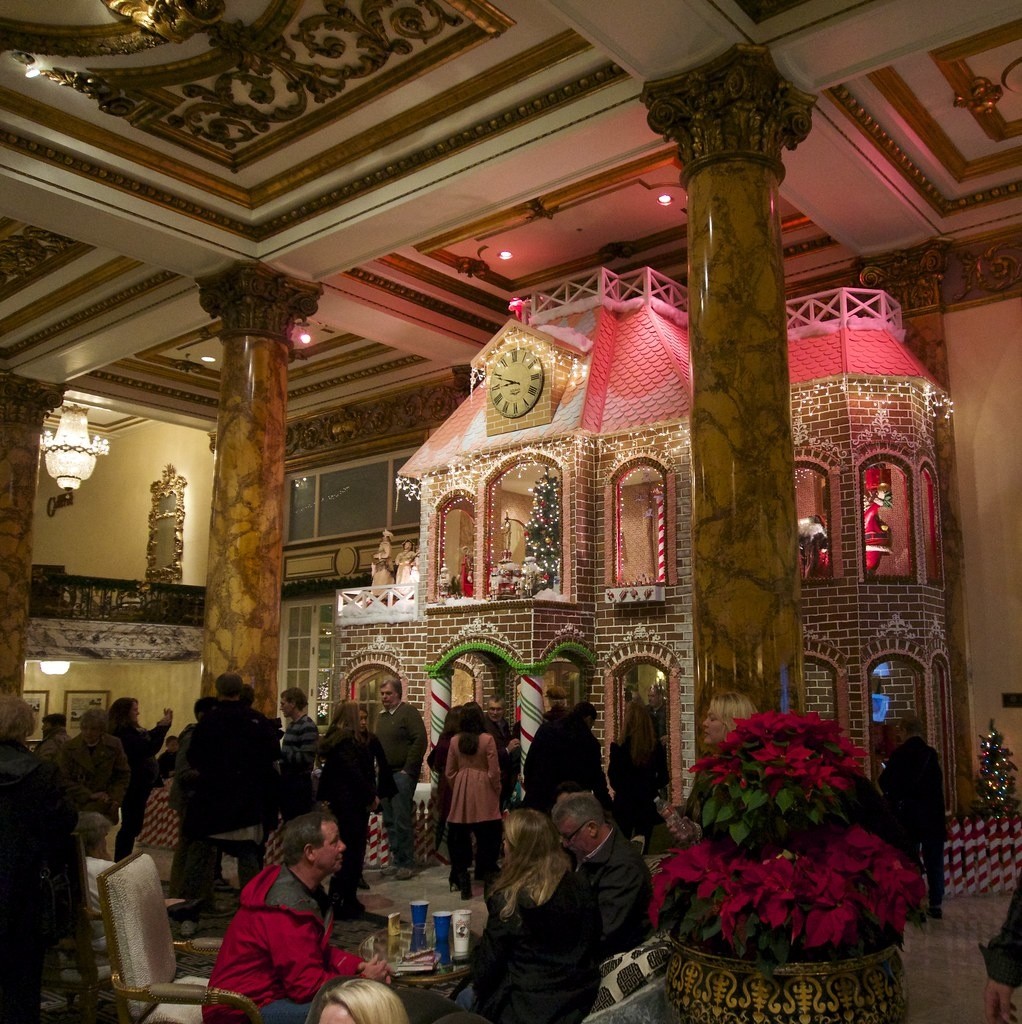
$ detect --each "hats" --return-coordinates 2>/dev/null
[545,686,567,699]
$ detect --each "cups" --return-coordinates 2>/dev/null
[407,927,428,953]
[432,911,451,941]
[409,900,429,927]
[451,909,472,952]
[434,941,448,964]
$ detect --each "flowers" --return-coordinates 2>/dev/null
[649,709,930,968]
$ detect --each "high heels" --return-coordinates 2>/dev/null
[448,875,460,892]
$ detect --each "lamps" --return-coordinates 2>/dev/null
[42,405,111,500]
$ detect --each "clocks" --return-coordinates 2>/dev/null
[489,347,544,418]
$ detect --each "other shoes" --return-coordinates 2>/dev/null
[906,910,926,922]
[167,893,215,918]
[462,889,472,899]
[357,877,371,889]
[927,907,943,918]
[380,865,417,880]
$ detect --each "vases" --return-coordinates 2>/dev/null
[666,921,910,1024]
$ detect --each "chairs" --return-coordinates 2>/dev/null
[580,972,668,1024]
[41,833,264,1024]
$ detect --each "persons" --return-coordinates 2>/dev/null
[0,678,759,1024]
[395,538,416,583]
[877,714,947,923]
[978,865,1022,1024]
[371,529,395,600]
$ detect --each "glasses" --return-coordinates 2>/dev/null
[559,820,591,840]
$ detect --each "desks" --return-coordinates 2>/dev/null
[361,922,482,1002]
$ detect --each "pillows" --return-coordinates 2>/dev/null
[589,929,677,1017]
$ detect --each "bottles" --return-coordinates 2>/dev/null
[654,797,693,836]
